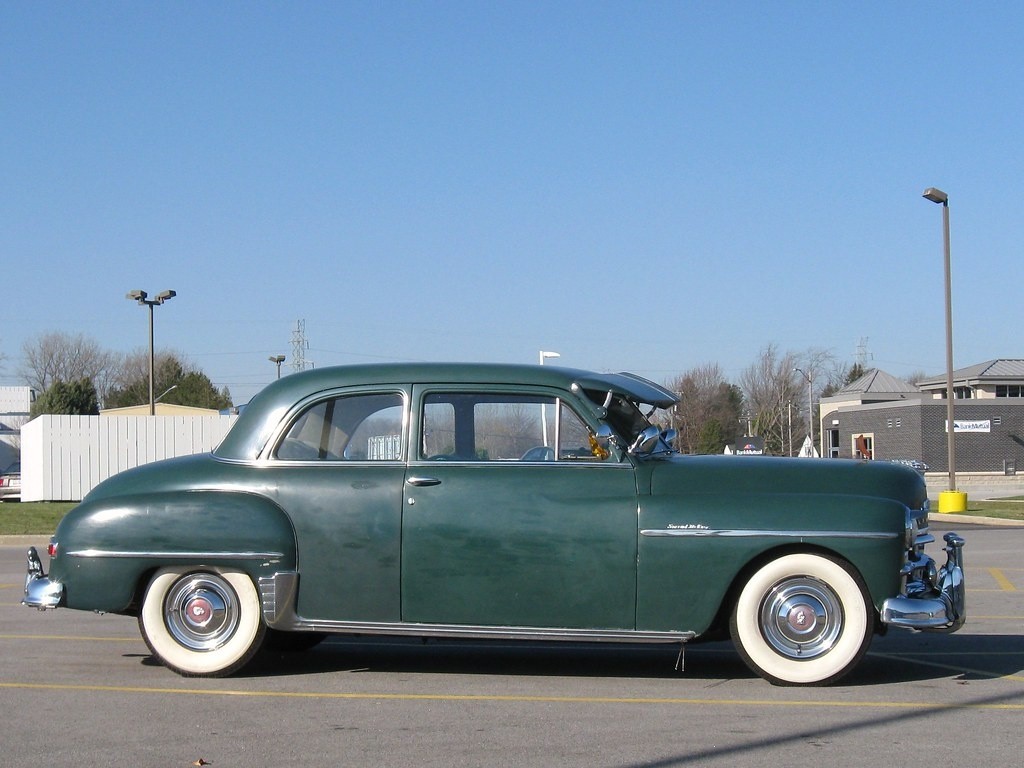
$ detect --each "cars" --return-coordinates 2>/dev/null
[22,360,970,689]
[0,460,21,502]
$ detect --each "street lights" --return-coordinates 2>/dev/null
[126,289,178,414]
[921,186,956,491]
[791,368,814,457]
[537,350,563,459]
[268,355,286,379]
